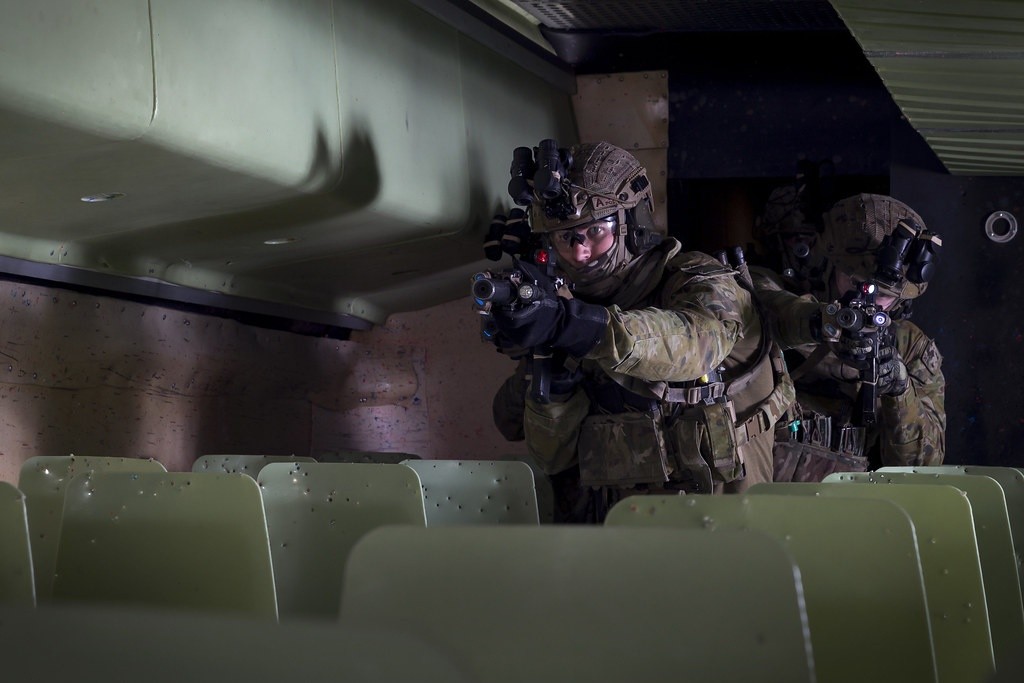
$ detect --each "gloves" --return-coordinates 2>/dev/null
[493,262,612,360]
[808,289,908,396]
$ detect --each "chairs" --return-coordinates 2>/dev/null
[0,456,1024,683]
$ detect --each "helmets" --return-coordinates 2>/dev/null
[815,193,932,300]
[529,142,655,233]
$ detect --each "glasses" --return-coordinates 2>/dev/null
[834,254,906,298]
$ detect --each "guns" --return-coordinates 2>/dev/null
[467,240,569,407]
[833,279,899,419]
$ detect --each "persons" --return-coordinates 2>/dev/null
[491,141,773,526]
[739,193,947,469]
